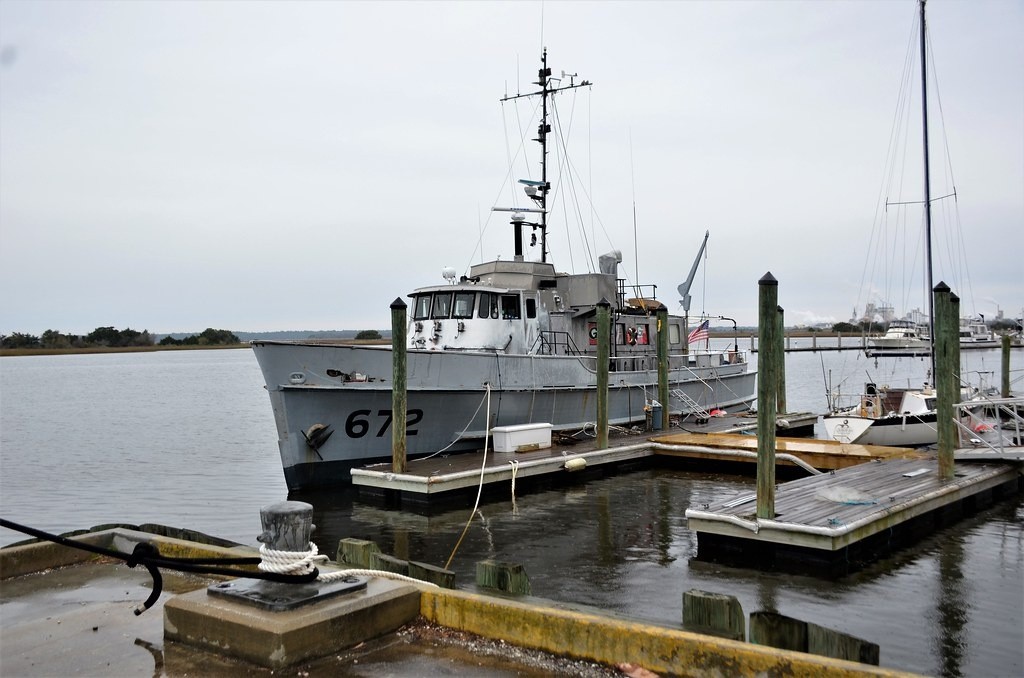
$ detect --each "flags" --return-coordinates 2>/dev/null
[688,319,709,343]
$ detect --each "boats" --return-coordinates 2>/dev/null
[997,330,1024,348]
[251,2,758,498]
[916,313,1000,349]
[868,306,935,350]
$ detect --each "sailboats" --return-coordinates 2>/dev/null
[807,0,1024,448]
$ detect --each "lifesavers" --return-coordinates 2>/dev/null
[626,327,638,346]
